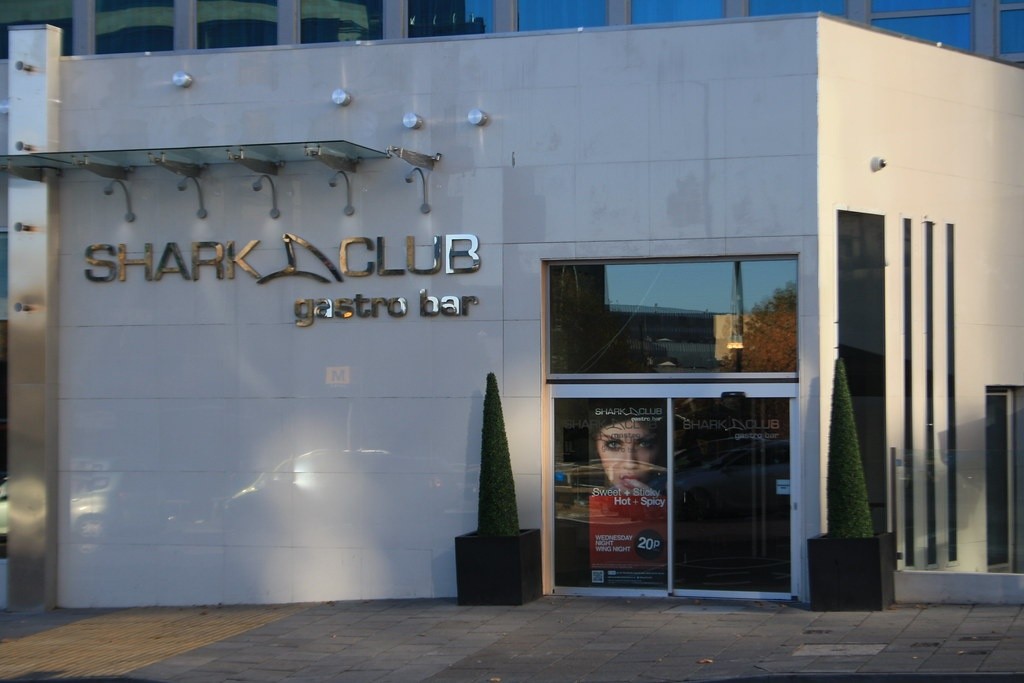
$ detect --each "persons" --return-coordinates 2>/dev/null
[590,397,666,495]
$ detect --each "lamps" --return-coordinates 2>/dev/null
[100,164,432,223]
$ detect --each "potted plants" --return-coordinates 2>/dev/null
[807,359,895,612]
[454,372,542,605]
